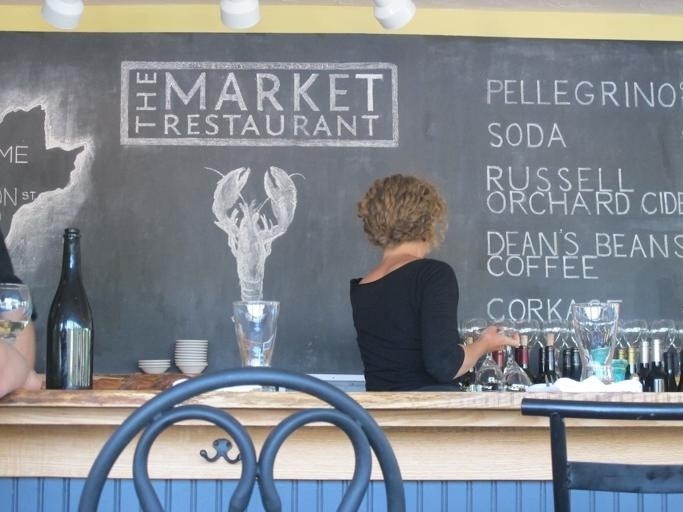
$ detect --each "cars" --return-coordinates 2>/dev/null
[232,300,280,367]
[0,283,34,343]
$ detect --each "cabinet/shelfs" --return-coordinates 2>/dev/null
[44,228,96,390]
[0,390,682,511]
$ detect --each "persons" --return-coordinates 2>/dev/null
[344,174,523,393]
[0,225,42,406]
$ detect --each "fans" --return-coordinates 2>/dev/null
[0,31,683,374]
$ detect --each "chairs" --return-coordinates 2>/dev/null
[520,398,682,512]
[78,370,405,511]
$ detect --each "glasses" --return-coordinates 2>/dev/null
[40,0,84,30]
[220,0,259,29]
[372,0,415,29]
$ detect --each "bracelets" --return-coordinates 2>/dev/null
[138,336,209,378]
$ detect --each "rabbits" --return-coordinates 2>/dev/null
[205,165,307,320]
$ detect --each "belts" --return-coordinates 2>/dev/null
[459,300,682,396]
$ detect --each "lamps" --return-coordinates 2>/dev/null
[372,0,415,29]
[220,0,259,29]
[40,0,84,30]
